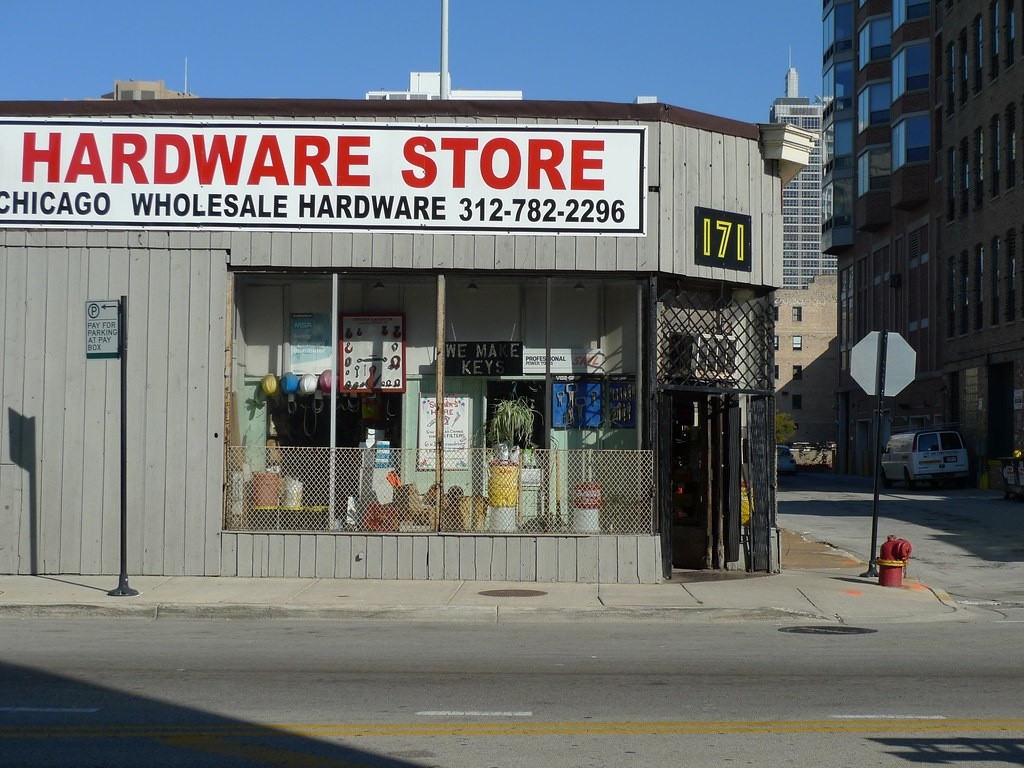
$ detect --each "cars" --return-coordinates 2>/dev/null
[777,445,796,476]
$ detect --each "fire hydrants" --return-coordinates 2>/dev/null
[876,534,913,588]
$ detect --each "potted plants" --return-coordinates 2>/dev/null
[687,424,702,441]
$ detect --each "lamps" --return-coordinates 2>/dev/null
[574,282,586,291]
[373,282,385,290]
[464,278,479,291]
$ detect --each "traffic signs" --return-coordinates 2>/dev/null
[85,299,120,359]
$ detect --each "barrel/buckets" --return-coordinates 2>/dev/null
[489,460,518,507]
[575,509,600,533]
[253,471,280,506]
[285,481,303,506]
[490,507,517,533]
[574,483,601,509]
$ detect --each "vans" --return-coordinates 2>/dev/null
[879,429,971,491]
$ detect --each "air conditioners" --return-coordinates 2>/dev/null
[670,330,740,382]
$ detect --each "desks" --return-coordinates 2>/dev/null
[255,505,330,530]
